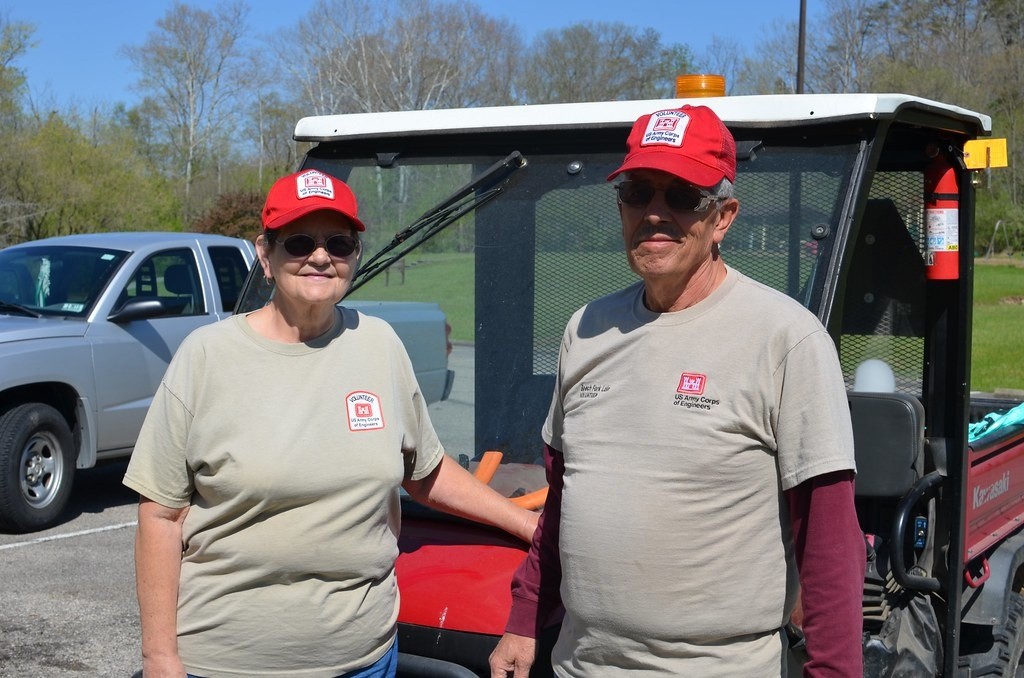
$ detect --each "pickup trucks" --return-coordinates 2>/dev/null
[0,229,456,535]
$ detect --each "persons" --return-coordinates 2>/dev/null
[119,169,542,678]
[489,105,865,678]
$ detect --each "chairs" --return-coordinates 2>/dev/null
[88,279,129,315]
[153,265,196,315]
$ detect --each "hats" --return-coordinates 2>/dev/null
[607,103,737,187]
[262,168,365,232]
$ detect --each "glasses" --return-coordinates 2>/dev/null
[274,233,360,258]
[614,178,728,216]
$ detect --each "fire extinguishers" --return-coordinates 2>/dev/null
[924,127,962,281]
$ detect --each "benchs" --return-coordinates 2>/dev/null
[470,373,928,629]
